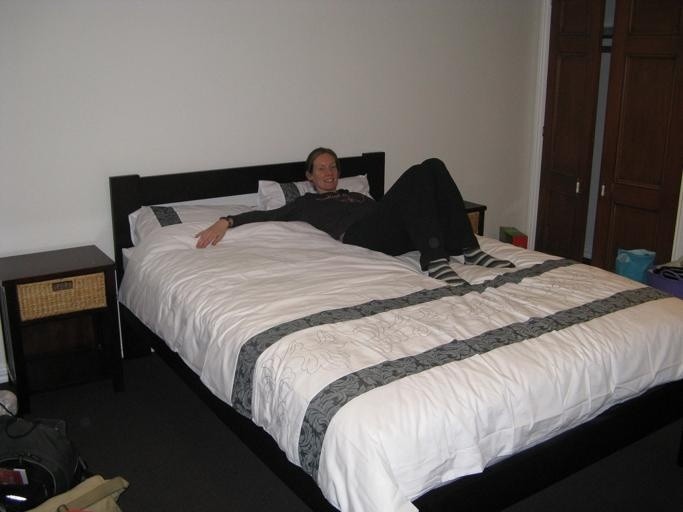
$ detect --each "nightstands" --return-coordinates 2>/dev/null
[0,245,126,415]
[463,199,486,237]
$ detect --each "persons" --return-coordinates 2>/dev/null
[192,148,516,292]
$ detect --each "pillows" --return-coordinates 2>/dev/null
[128,172,374,250]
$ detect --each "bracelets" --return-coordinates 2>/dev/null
[218,216,232,229]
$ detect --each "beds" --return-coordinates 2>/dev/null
[106,152,683,511]
